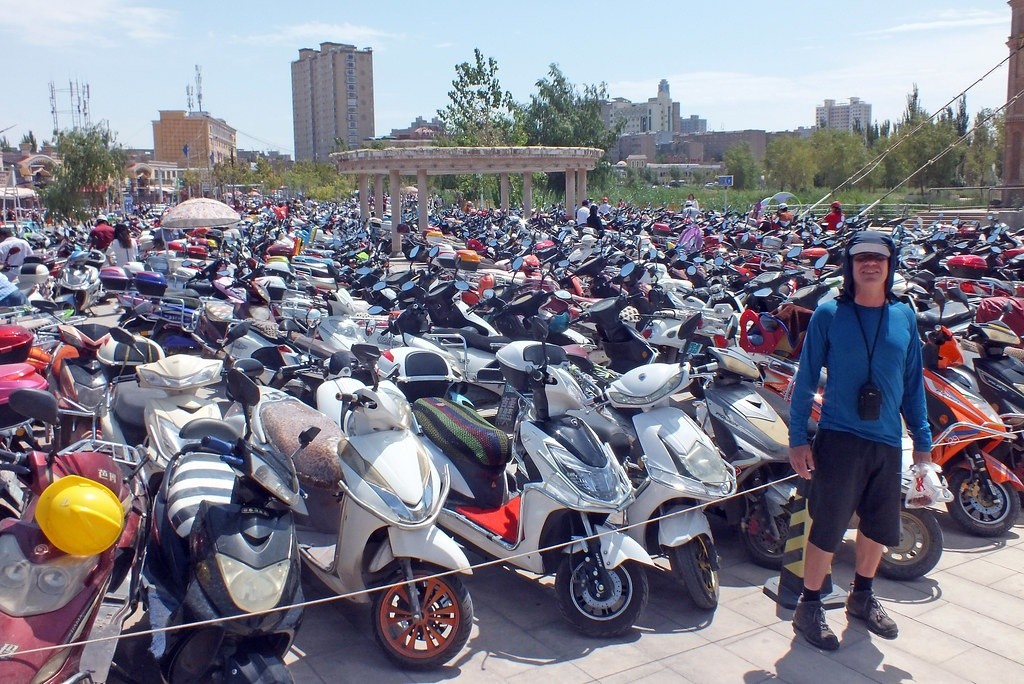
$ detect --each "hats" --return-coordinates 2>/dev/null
[603,198,608,202]
[685,200,692,205]
[777,203,788,209]
[848,231,891,258]
[829,201,841,208]
[96,215,108,221]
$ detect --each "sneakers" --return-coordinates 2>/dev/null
[792,597,840,649]
[846,582,898,636]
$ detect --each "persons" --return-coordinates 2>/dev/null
[1,228,35,282]
[84,213,115,255]
[152,225,193,253]
[683,200,699,223]
[782,230,935,650]
[815,200,845,231]
[685,193,699,212]
[774,203,793,225]
[588,205,606,234]
[574,200,588,228]
[226,191,565,233]
[617,199,626,208]
[104,223,140,268]
[596,198,611,216]
[0,200,164,237]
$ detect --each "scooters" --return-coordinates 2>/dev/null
[0,192,1023,684]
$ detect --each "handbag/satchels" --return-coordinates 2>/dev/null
[903,461,942,509]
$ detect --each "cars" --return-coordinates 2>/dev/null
[651,180,718,189]
